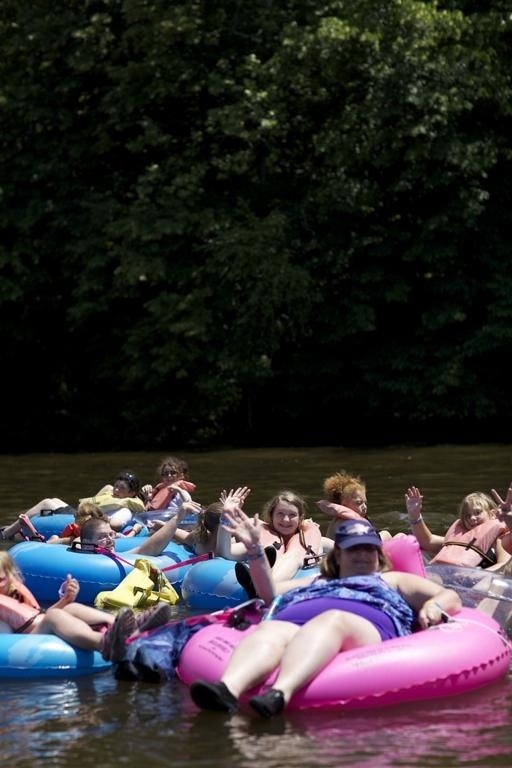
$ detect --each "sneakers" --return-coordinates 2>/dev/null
[190,679,238,715]
[264,546,276,568]
[235,562,257,598]
[135,603,170,631]
[247,691,284,717]
[101,608,136,660]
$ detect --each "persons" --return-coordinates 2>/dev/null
[1,458,511,721]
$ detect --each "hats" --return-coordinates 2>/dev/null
[334,520,381,550]
